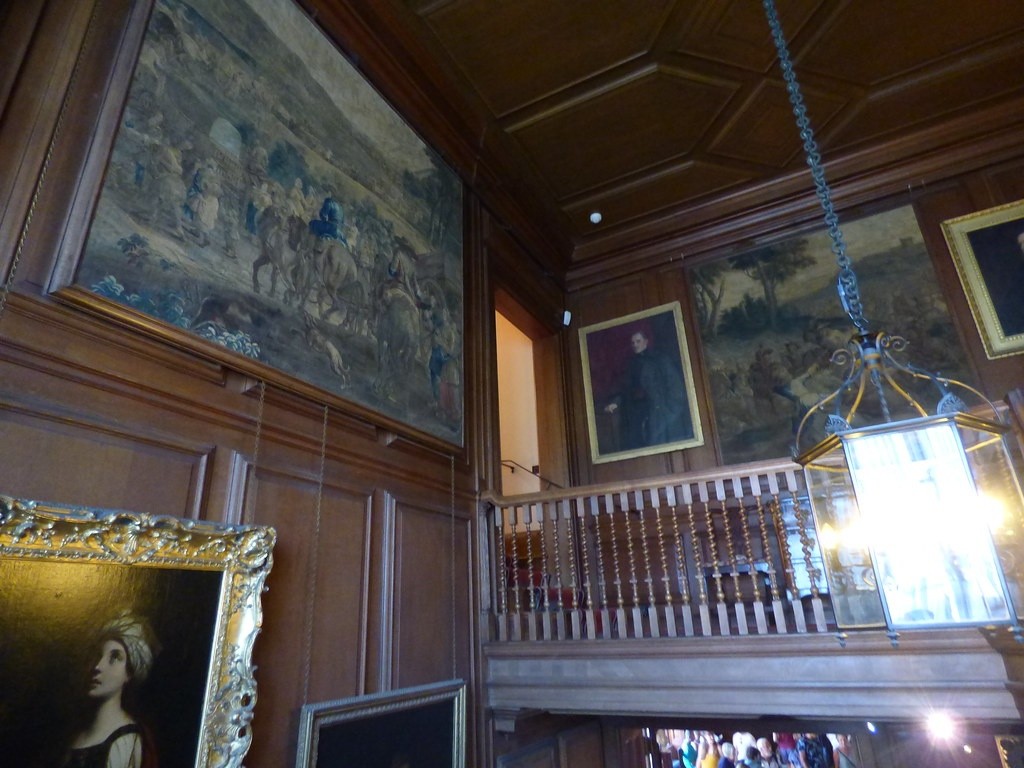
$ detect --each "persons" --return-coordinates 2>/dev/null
[654,727,863,768]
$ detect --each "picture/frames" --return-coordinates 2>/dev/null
[45,0,479,474]
[940,198,1024,359]
[577,300,706,465]
[296,677,467,768]
[0,492,278,768]
